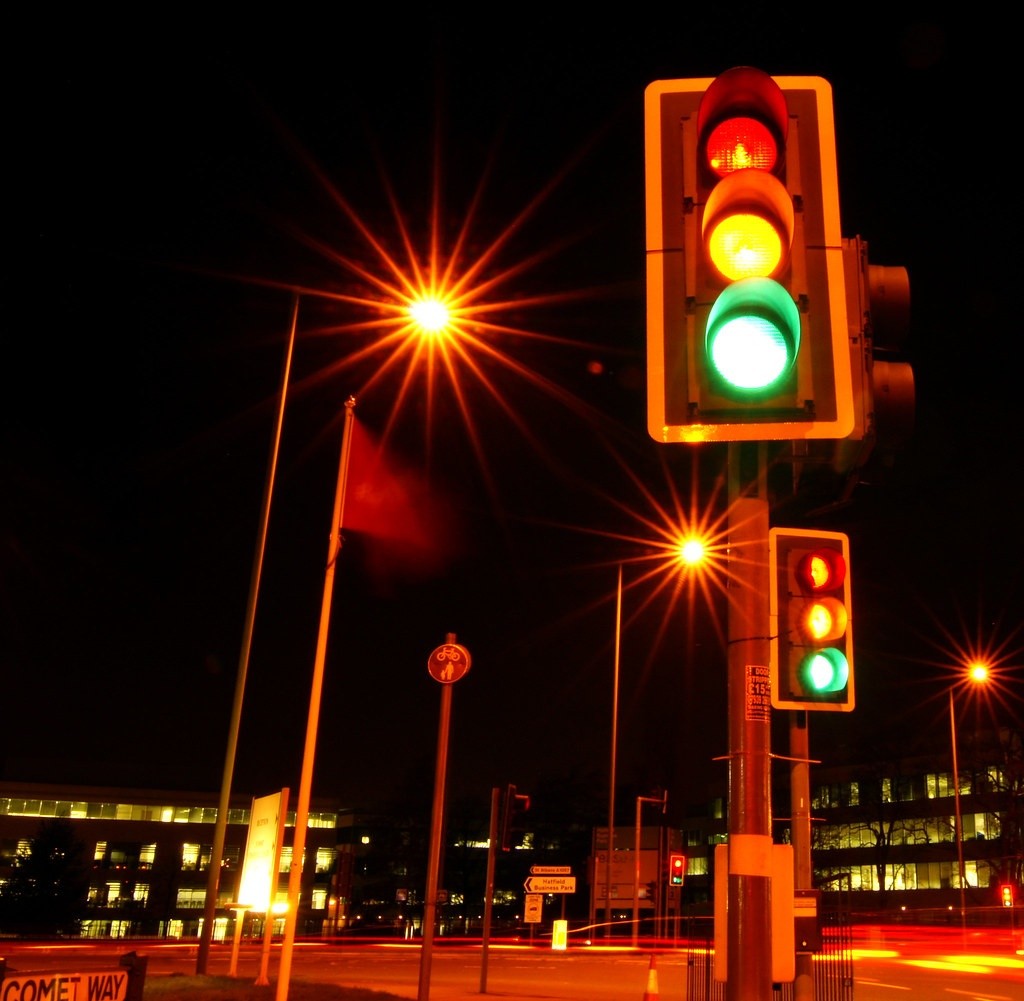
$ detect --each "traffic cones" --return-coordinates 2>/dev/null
[641,952,666,1001]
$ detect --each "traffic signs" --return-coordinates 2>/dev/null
[523,876,576,895]
[530,865,573,876]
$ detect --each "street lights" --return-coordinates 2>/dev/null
[191,282,467,977]
[944,658,1001,930]
[606,533,709,945]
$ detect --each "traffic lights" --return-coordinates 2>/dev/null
[641,67,869,447]
[1001,884,1014,909]
[831,235,927,442]
[669,855,688,889]
[766,528,869,726]
